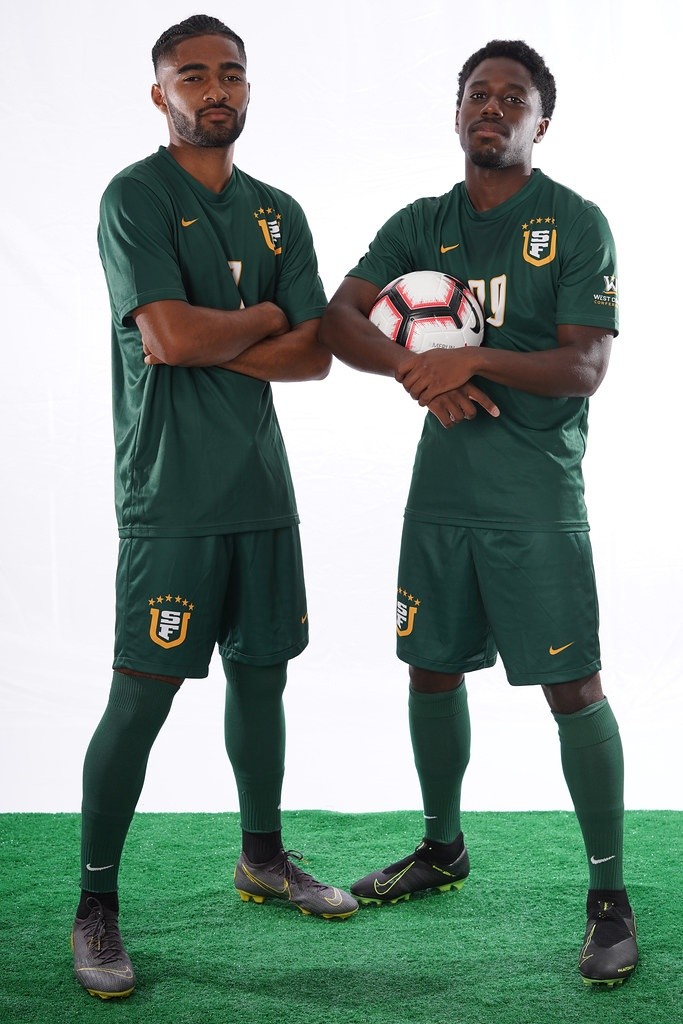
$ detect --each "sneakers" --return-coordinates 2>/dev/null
[233,841,472,919]
[577,897,641,987]
[70,907,137,1002]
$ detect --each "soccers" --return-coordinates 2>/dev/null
[367,268,486,353]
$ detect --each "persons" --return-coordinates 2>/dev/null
[65,11,360,999]
[316,39,640,986]
